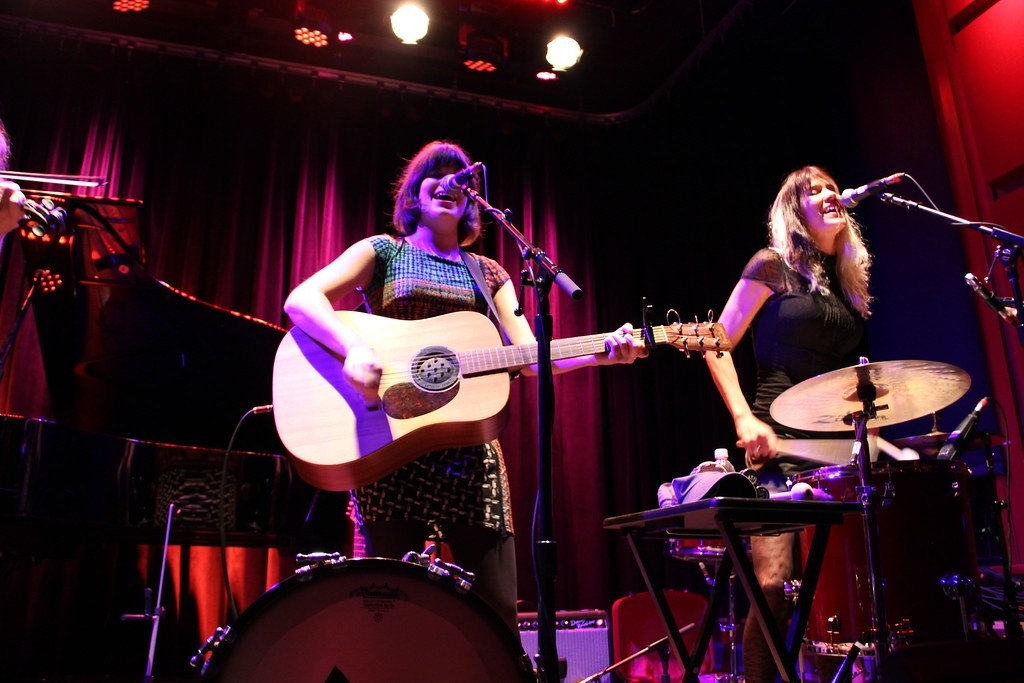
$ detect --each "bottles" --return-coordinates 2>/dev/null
[715,448,736,472]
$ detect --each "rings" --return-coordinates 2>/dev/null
[755,445,760,453]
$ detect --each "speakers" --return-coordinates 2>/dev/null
[518,608,614,683]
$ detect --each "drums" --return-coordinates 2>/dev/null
[194,556,538,683]
[786,458,985,658]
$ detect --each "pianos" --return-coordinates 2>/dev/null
[0,196,352,682]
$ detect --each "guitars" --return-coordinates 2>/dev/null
[271,308,731,492]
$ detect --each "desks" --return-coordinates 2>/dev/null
[598,496,863,683]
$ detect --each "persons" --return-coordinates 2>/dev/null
[283,141,651,662]
[0,124,26,234]
[705,165,879,683]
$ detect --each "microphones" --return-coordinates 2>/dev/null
[442,162,484,194]
[842,172,907,208]
[938,396,991,461]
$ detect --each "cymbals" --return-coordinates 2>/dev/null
[769,359,971,433]
